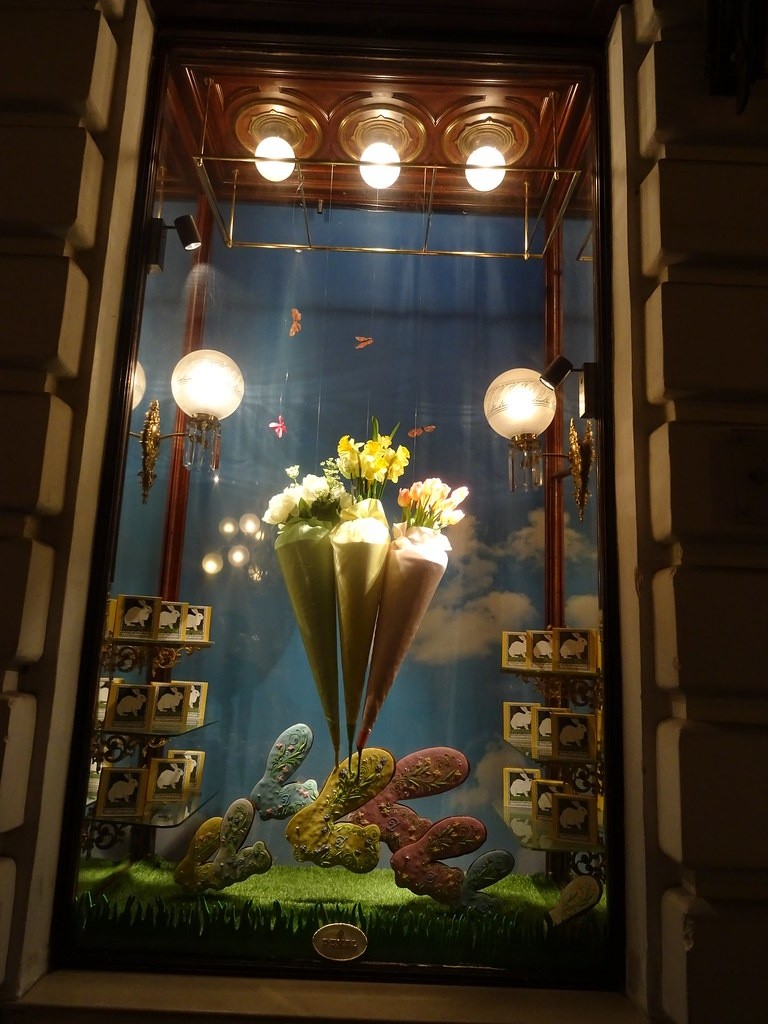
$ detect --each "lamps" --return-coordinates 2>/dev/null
[540,357,602,419]
[139,349,244,505]
[465,139,506,193]
[254,130,296,182]
[483,368,585,521]
[147,213,202,273]
[358,134,401,189]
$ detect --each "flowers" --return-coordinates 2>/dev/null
[335,415,411,505]
[397,477,469,530]
[261,457,357,533]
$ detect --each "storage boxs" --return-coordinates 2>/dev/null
[168,750,206,793]
[502,630,527,669]
[104,683,156,731]
[145,757,191,801]
[552,792,598,846]
[172,680,209,720]
[551,710,597,761]
[552,626,598,674]
[531,779,572,824]
[157,600,189,640]
[186,605,211,641]
[94,767,150,817]
[530,705,571,749]
[112,594,162,640]
[502,701,541,741]
[525,629,554,672]
[503,767,540,807]
[150,681,191,726]
